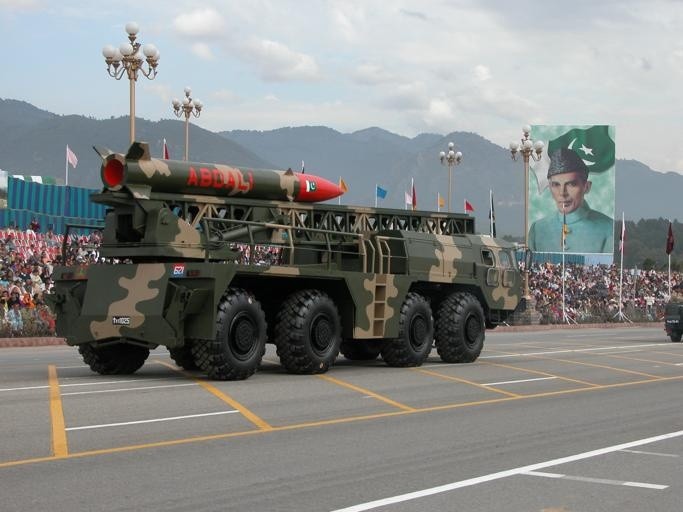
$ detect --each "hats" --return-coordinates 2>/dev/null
[547,149,589,178]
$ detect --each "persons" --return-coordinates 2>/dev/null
[0,216,134,337]
[224,241,285,266]
[516,259,683,322]
[527,147,614,254]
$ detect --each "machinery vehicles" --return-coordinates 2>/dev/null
[664,281,683,341]
[42,140,524,382]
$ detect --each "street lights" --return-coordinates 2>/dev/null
[510,125,543,297]
[439,141,462,212]
[171,86,202,162]
[102,22,159,144]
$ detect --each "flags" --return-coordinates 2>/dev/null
[377,187,388,199]
[301,165,306,174]
[68,149,79,168]
[665,222,674,255]
[558,206,568,250]
[529,125,615,196]
[164,141,170,160]
[488,196,498,239]
[406,184,417,210]
[465,203,475,212]
[438,195,446,208]
[339,179,348,194]
[618,217,627,253]
